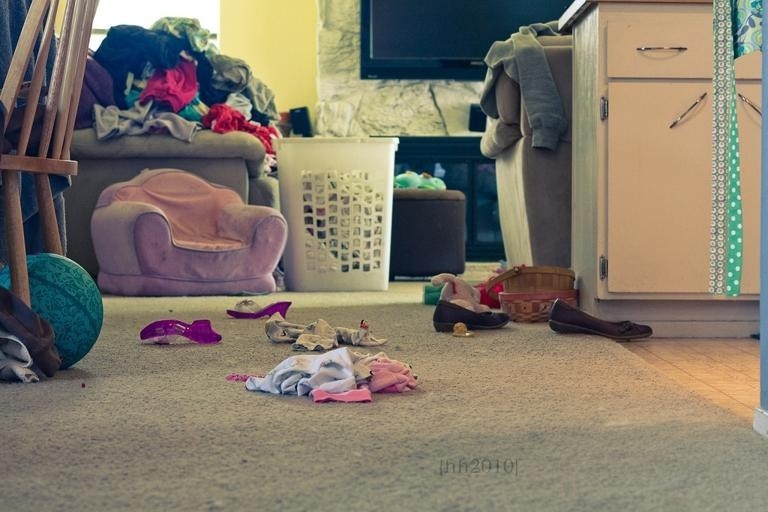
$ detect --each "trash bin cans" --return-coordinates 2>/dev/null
[270,137,400,293]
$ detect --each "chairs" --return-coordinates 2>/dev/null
[3,0,158,314]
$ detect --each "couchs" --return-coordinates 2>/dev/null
[44,30,280,296]
[475,26,572,268]
[92,168,286,294]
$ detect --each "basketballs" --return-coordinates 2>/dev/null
[2,253,103,368]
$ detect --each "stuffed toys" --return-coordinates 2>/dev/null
[430,272,491,313]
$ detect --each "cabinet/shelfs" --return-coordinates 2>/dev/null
[553,1,763,338]
[368,136,503,265]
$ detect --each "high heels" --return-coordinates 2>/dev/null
[226,299,293,322]
[139,318,222,345]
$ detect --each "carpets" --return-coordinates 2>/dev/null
[0,301,768,512]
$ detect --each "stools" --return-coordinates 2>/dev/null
[391,183,467,281]
[472,151,479,153]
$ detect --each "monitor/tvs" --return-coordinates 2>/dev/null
[360,0,575,84]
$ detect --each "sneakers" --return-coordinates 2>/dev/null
[433,299,510,334]
[548,298,653,343]
